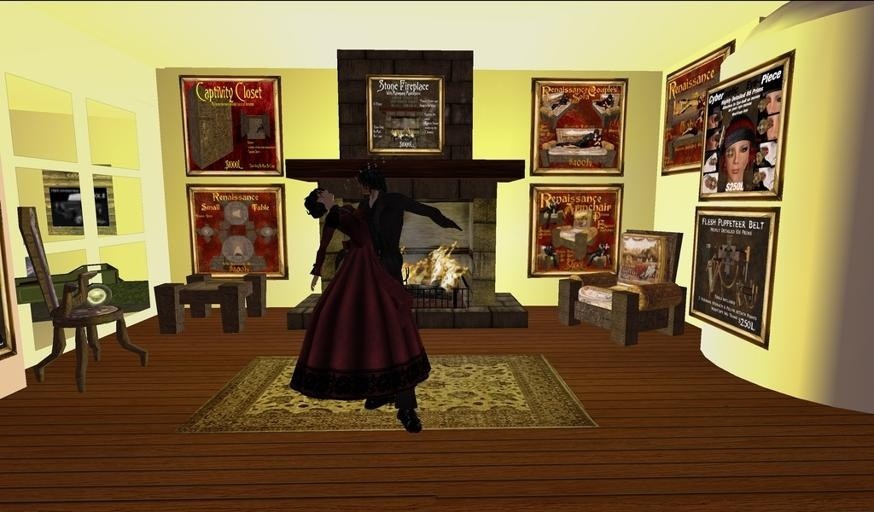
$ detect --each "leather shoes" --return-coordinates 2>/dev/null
[396,407,421,433]
